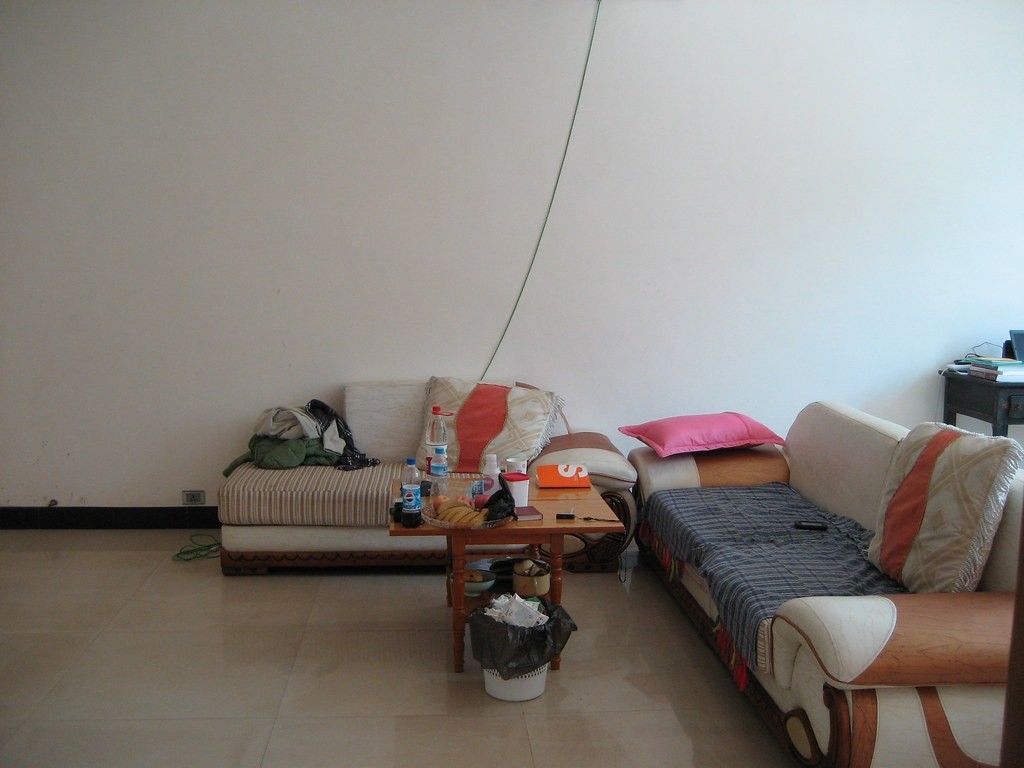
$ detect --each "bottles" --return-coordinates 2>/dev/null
[430,448,448,504]
[402,458,421,528]
[483,454,501,496]
[426,406,453,482]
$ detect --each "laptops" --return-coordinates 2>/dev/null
[1010,330,1024,363]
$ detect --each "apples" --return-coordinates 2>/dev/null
[464,569,483,581]
[433,496,450,511]
[455,495,471,505]
[514,557,533,574]
[475,495,490,509]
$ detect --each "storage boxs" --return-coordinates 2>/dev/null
[502,472,529,507]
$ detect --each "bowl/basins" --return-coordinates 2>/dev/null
[449,569,496,597]
[514,561,550,595]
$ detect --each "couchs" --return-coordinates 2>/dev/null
[618,400,1024,768]
[218,382,638,576]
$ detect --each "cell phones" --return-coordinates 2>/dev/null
[795,519,828,531]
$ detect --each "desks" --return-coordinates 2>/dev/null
[390,476,625,673]
[938,370,1024,438]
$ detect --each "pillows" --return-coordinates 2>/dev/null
[618,412,787,457]
[415,376,565,473]
[868,422,1024,594]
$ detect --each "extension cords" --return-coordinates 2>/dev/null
[947,363,971,371]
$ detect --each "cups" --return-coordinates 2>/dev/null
[502,473,529,507]
[506,458,527,474]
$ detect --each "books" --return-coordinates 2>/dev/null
[968,357,1024,382]
[535,465,592,488]
[514,506,543,521]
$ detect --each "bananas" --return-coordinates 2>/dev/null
[436,499,489,523]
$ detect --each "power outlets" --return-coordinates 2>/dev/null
[183,490,205,505]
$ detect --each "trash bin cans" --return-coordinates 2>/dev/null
[476,598,560,701]
